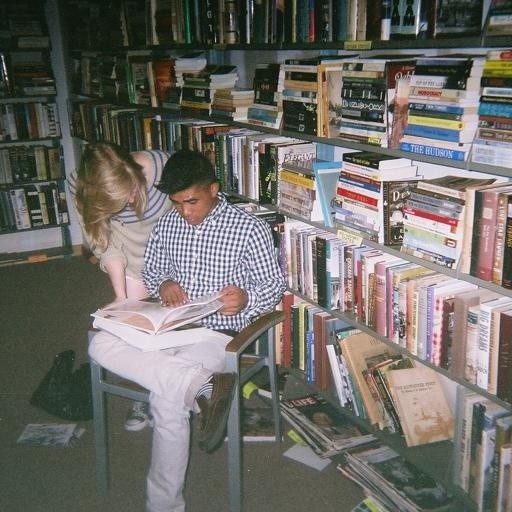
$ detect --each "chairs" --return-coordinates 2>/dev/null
[88,229,288,511]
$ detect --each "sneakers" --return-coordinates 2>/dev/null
[195,372,238,452]
[124,401,149,432]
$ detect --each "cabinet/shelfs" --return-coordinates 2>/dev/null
[0,0,69,250]
[60,0,512,512]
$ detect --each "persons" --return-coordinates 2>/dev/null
[88,148,287,512]
[73,139,174,435]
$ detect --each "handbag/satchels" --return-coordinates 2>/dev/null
[29,348,93,419]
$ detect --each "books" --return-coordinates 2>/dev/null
[1,1,73,234]
[92,291,227,352]
[60,2,511,287]
[224,195,512,512]
